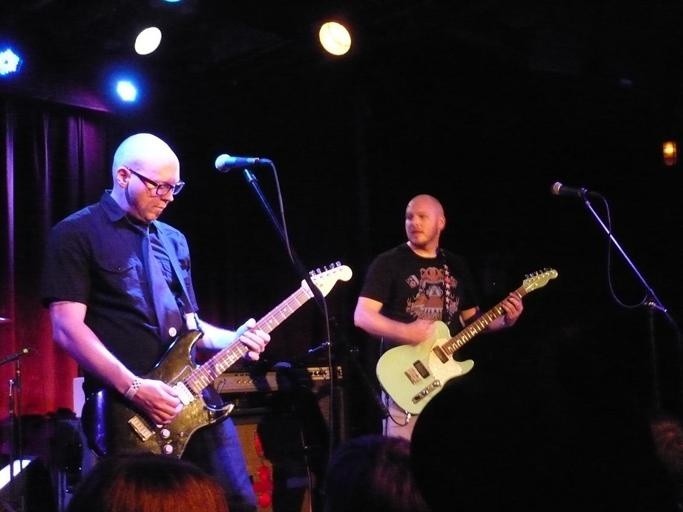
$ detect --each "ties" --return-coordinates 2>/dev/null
[124,219,183,350]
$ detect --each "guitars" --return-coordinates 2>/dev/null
[82,262,353,478]
[375,269,559,416]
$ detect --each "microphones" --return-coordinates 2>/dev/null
[551,180,601,200]
[214,153,272,174]
[0,347,32,367]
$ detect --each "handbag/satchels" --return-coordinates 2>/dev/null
[80,388,110,456]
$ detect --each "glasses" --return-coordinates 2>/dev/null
[128,169,185,197]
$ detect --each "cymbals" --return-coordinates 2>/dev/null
[0,413,65,456]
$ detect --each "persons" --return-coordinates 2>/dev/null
[48,132,271,511]
[306,438,427,512]
[355,194,524,437]
[64,455,232,512]
